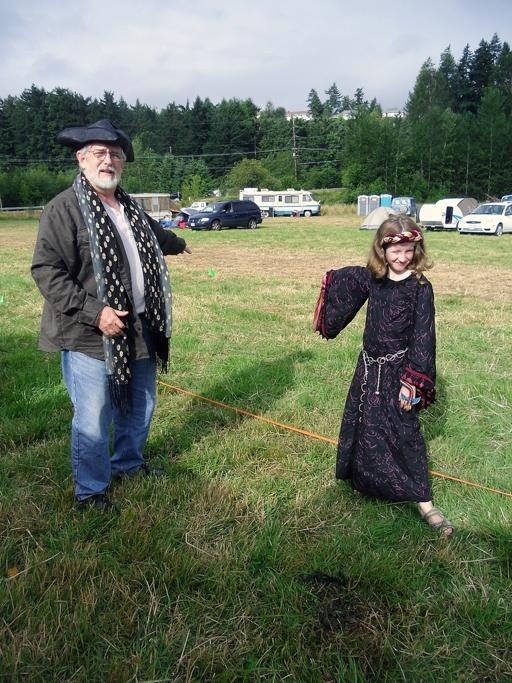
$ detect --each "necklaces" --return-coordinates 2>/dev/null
[312,214,456,538]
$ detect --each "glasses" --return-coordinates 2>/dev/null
[87,149,123,161]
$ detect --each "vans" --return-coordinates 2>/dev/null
[239,188,321,218]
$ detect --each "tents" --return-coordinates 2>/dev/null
[360,206,396,229]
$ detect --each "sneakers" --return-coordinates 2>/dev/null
[120,459,168,477]
[79,494,120,518]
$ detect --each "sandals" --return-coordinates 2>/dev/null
[419,507,454,538]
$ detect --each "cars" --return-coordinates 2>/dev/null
[188,200,262,231]
[457,195,512,237]
[170,193,182,199]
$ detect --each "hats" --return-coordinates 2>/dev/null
[56,119,135,163]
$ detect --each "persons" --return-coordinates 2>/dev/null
[29,119,192,519]
[159,216,188,228]
